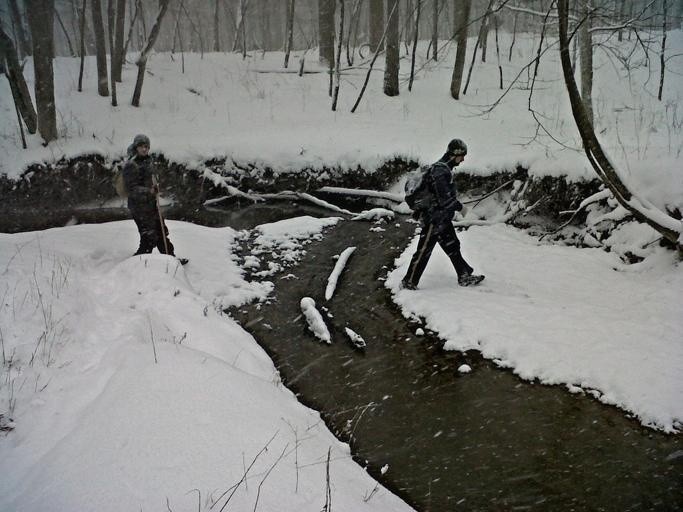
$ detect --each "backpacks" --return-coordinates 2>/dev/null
[404,167,432,212]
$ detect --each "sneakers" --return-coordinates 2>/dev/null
[401,280,420,290]
[458,275,486,287]
[178,258,189,265]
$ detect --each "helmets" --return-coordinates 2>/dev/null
[134,134,150,147]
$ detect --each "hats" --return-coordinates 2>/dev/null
[447,139,467,155]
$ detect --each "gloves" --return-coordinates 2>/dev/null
[461,205,468,218]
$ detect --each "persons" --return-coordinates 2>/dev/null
[123,135,188,266]
[399,139,486,290]
